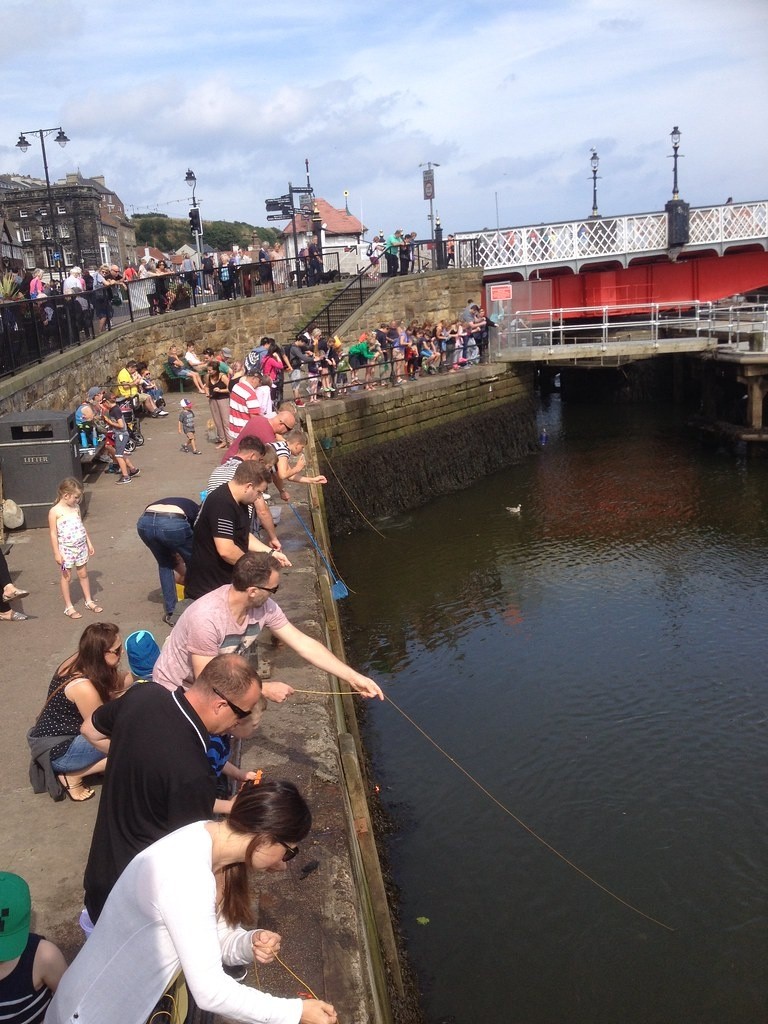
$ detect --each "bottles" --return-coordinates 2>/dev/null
[92,428,98,447]
[80,429,88,447]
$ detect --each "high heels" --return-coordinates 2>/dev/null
[57,773,95,802]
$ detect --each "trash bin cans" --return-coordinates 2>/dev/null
[0,409,87,529]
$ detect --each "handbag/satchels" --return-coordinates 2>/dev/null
[95,284,106,300]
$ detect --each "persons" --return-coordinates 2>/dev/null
[0,872,67,1024]
[150,552,384,703]
[219,411,296,501]
[244,298,491,407]
[265,430,328,485]
[26,622,139,802]
[1,240,326,378]
[260,445,280,473]
[228,369,271,500]
[44,781,338,1023]
[137,497,201,626]
[206,701,264,783]
[724,197,734,238]
[183,460,293,600]
[208,435,266,519]
[366,222,591,281]
[80,654,264,981]
[47,474,102,618]
[0,546,29,620]
[75,340,246,485]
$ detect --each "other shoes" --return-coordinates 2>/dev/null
[2,589,29,602]
[0,610,28,621]
[163,614,175,627]
[292,358,472,408]
[105,390,227,484]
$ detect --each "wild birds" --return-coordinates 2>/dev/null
[505,504,521,513]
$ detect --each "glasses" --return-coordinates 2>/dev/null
[104,581,299,861]
[113,270,120,273]
[280,420,292,432]
[158,263,165,266]
[145,373,150,376]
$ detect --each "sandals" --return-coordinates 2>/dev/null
[64,605,82,619]
[84,600,102,612]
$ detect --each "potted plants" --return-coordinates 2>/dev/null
[168,282,191,310]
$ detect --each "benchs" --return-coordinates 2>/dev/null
[163,358,207,393]
[75,405,105,473]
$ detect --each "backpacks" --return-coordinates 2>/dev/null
[366,243,378,256]
[299,248,309,262]
[285,344,298,364]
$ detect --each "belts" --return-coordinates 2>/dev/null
[144,512,186,519]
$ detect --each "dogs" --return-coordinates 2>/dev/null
[206,418,219,440]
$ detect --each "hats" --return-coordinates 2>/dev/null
[89,386,104,400]
[246,367,277,388]
[0,872,32,962]
[126,631,161,676]
[180,399,192,407]
[221,347,232,357]
[99,392,116,404]
